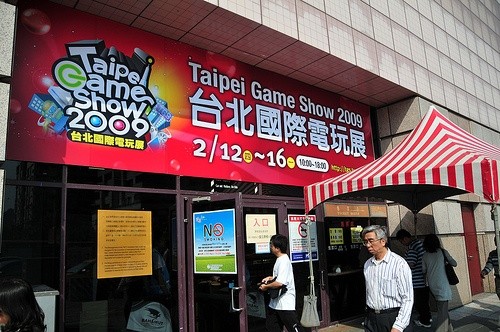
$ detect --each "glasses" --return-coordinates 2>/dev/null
[399,236,405,244]
[362,238,383,246]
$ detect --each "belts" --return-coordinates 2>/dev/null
[367,305,401,314]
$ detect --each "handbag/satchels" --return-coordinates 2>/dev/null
[441,249,459,285]
[300,284,320,327]
[258,277,287,299]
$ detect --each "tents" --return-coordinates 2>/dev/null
[303,107,500,332]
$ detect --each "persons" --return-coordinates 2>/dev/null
[396,228,432,332]
[0,279,47,332]
[89,242,271,332]
[362,225,414,332]
[480,235,500,300]
[422,232,457,332]
[258,234,303,332]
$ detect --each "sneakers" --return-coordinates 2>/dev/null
[413,320,431,328]
